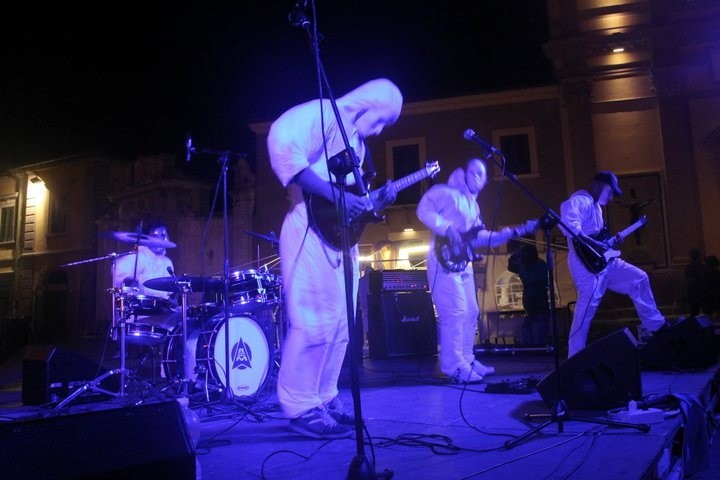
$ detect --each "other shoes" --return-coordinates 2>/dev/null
[467,359,494,376]
[440,360,483,383]
[651,316,687,336]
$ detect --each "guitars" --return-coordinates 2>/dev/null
[573,213,648,274]
[435,217,539,272]
[305,161,441,253]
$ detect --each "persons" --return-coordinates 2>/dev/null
[416,158,514,384]
[556,171,686,360]
[266,78,404,441]
[113,221,201,382]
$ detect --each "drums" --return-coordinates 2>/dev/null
[162,308,271,398]
[116,294,178,347]
[257,271,282,306]
[223,269,266,310]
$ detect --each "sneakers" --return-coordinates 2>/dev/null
[324,398,367,429]
[289,404,354,439]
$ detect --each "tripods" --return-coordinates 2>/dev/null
[187,148,266,421]
[497,160,651,449]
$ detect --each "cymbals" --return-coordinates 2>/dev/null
[145,274,225,295]
[100,230,178,250]
[242,229,279,243]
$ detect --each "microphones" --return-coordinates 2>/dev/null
[185,136,192,161]
[288,0,307,26]
[463,129,503,156]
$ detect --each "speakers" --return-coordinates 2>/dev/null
[0,401,197,480]
[642,317,716,370]
[535,327,643,411]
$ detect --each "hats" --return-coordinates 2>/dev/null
[593,171,623,196]
